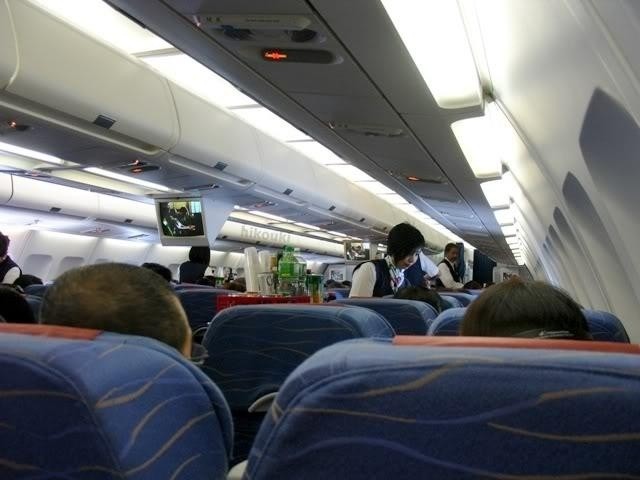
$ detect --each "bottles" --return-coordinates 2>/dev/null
[214,265,225,288]
[277,246,307,297]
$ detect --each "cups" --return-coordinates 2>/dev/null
[243,246,275,294]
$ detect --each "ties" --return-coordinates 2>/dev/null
[454,264,457,274]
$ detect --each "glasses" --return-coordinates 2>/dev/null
[184,342,211,366]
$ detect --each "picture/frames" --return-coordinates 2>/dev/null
[327,268,346,283]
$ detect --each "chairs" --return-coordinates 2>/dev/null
[16,296,52,323]
[177,289,231,326]
[427,305,632,346]
[453,291,472,305]
[330,296,435,334]
[0,323,235,480]
[437,293,461,312]
[201,303,395,472]
[242,334,638,480]
[25,283,56,296]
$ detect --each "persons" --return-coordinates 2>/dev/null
[437,243,465,290]
[458,276,595,342]
[347,223,425,299]
[402,250,439,290]
[175,206,191,225]
[392,284,443,317]
[463,281,481,290]
[158,202,199,237]
[0,232,248,364]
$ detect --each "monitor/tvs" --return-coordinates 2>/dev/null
[344,241,378,264]
[154,194,232,247]
